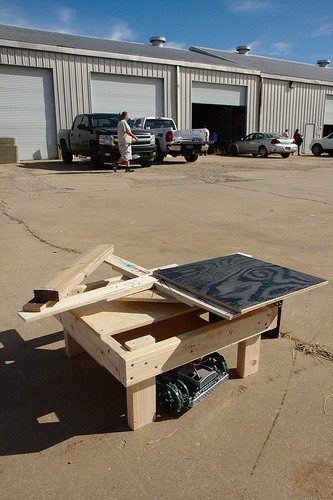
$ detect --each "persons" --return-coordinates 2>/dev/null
[113,112,140,172]
[294,128,303,156]
[283,129,289,139]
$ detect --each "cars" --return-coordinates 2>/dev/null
[227,132,297,158]
[309,132,333,156]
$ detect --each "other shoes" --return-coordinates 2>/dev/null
[113,162,117,172]
[126,168,135,172]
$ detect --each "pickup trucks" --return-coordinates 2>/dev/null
[132,117,214,162]
[58,112,156,167]
